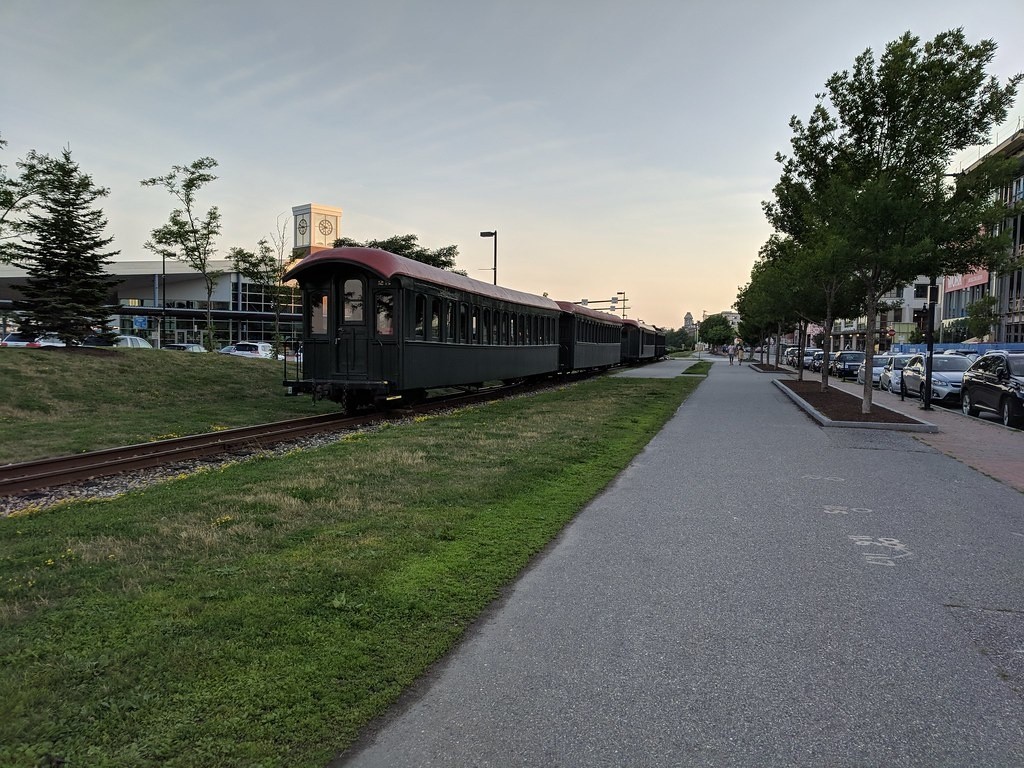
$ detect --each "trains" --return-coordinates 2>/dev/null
[282,251,668,419]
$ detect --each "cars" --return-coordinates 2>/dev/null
[162,343,208,352]
[217,346,235,355]
[856,355,893,384]
[782,344,836,372]
[741,345,768,352]
[881,355,918,394]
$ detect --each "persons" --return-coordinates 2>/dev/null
[728,342,736,366]
[738,345,744,366]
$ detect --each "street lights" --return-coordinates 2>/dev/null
[161,252,177,347]
[917,302,937,412]
[617,292,626,321]
[479,230,497,286]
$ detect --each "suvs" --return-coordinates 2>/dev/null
[82,335,152,349]
[962,349,1024,426]
[901,351,975,407]
[833,350,866,378]
[230,341,285,361]
[1,331,67,348]
